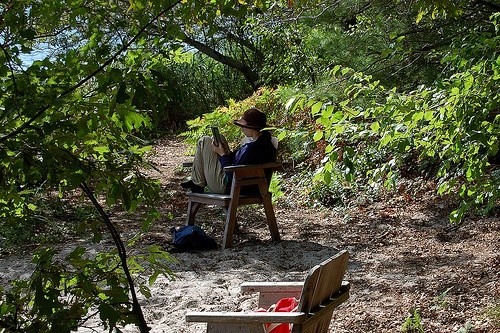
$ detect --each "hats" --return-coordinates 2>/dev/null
[234,108,267,130]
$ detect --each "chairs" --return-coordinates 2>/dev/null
[185,249,352,333]
[181,136,281,250]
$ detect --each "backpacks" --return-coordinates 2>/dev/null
[171,225,217,249]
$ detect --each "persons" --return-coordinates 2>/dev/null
[180,109,276,196]
